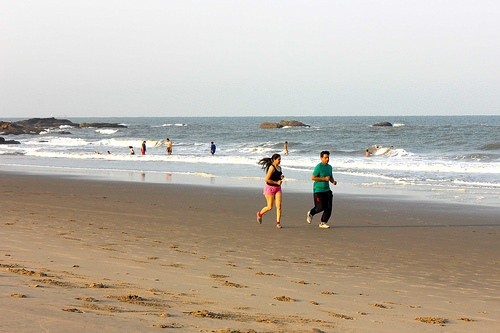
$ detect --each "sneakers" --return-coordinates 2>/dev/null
[275,220,282,229]
[306,211,313,224]
[318,222,330,229]
[257,212,262,224]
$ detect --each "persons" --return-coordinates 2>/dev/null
[306,151,337,228]
[129,146,134,155]
[141,141,146,155]
[167,138,172,155]
[257,154,284,229]
[283,141,288,155]
[366,149,369,157]
[211,142,216,154]
